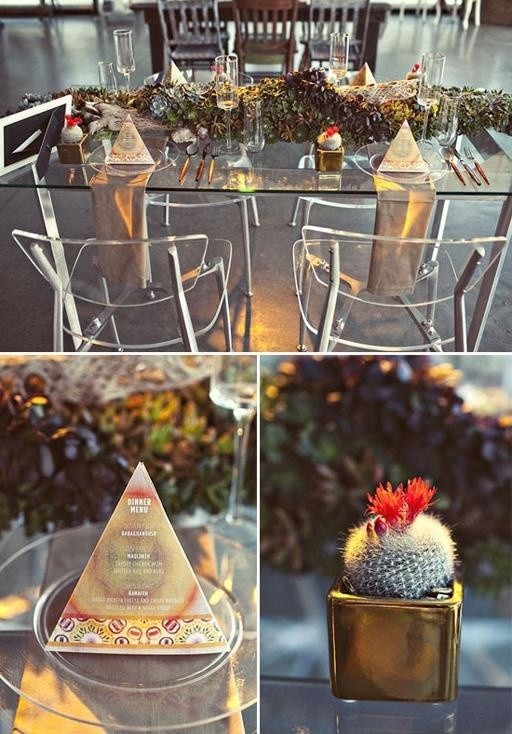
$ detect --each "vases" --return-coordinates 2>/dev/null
[318,148,343,172]
[327,577,463,702]
[57,135,90,164]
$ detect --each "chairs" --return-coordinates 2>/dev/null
[234,21,285,57]
[229,0,298,84]
[12,229,232,352]
[143,66,261,297]
[289,73,377,295]
[292,225,509,352]
[306,1,371,69]
[300,21,343,68]
[185,19,230,77]
[155,0,226,79]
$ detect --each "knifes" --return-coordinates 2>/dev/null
[454,145,481,185]
[195,141,210,183]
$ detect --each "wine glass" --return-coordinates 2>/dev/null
[215,55,241,156]
[208,355,259,551]
[418,51,446,152]
[112,27,136,92]
[329,31,349,88]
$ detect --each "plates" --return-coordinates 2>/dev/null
[0,516,255,732]
[87,135,181,177]
[351,140,452,188]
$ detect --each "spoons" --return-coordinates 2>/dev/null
[444,147,468,186]
[176,143,201,183]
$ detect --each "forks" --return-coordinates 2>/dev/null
[463,144,492,187]
[205,147,219,185]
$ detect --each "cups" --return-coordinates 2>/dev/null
[435,90,463,157]
[96,58,121,103]
[243,94,267,154]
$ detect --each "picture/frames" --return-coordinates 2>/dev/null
[1,92,71,176]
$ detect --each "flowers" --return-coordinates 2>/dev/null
[318,126,343,150]
[339,477,459,601]
[406,64,421,79]
[210,65,227,82]
[60,112,84,142]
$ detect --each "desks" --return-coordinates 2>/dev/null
[129,2,391,73]
